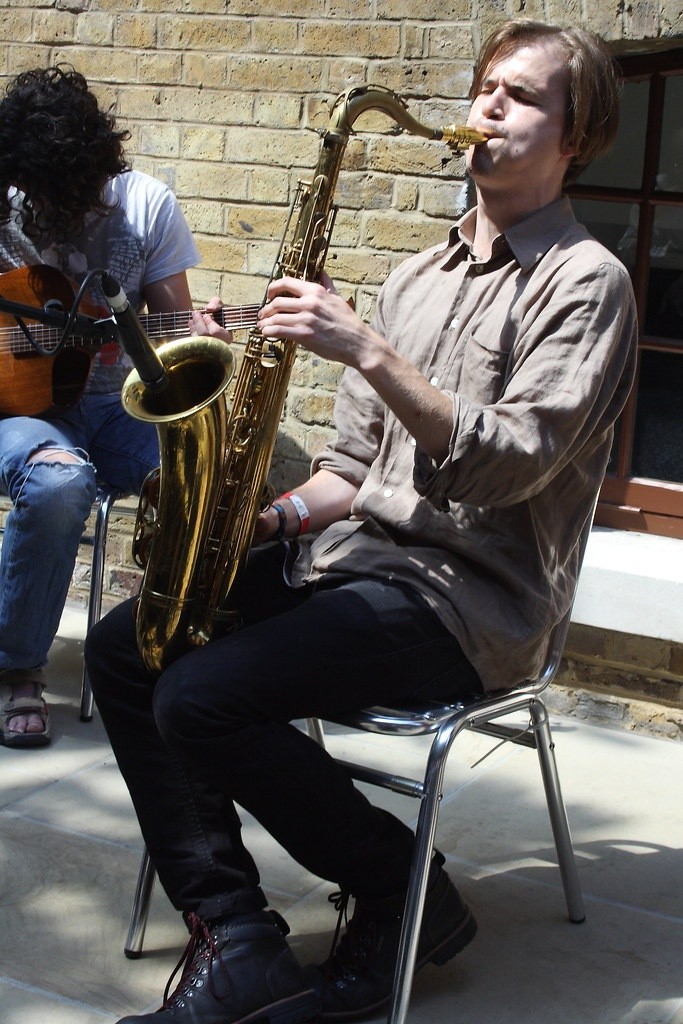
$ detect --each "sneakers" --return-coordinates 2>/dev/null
[119,903,323,1023]
[304,858,479,1022]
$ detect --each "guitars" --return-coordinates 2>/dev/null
[2,260,357,428]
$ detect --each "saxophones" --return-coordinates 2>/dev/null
[110,79,492,677]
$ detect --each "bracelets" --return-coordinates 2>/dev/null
[277,492,310,537]
[269,501,287,539]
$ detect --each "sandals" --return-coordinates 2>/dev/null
[0,666,52,749]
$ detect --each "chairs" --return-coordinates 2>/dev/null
[123,592,590,1024]
[0,482,133,725]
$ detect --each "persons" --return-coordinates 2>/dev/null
[83,17,639,1024]
[0,61,233,746]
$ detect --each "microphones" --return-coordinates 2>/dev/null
[102,273,170,392]
